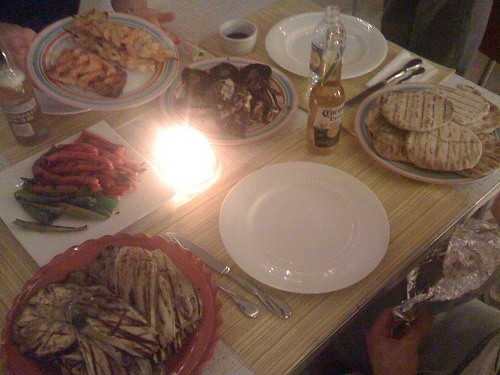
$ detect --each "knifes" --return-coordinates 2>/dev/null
[345,58,423,105]
[165,232,293,320]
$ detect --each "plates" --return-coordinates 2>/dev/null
[219,162,390,294]
[0,120,177,268]
[353,82,500,185]
[264,11,387,80]
[0,233,224,375]
[158,56,298,145]
[28,12,179,110]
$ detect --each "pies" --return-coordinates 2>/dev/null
[10,244,202,375]
[365,84,500,178]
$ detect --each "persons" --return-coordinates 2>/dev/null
[380,0,493,75]
[0,0,182,86]
[328,277,500,375]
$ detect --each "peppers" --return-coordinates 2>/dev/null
[13,188,118,225]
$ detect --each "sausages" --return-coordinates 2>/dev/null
[22,129,147,198]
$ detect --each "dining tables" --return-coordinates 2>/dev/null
[0,0,500,375]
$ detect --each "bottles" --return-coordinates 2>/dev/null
[305,27,345,156]
[0,39,49,146]
[304,5,343,104]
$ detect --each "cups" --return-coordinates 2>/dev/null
[219,19,258,57]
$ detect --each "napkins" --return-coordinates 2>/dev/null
[364,50,437,93]
